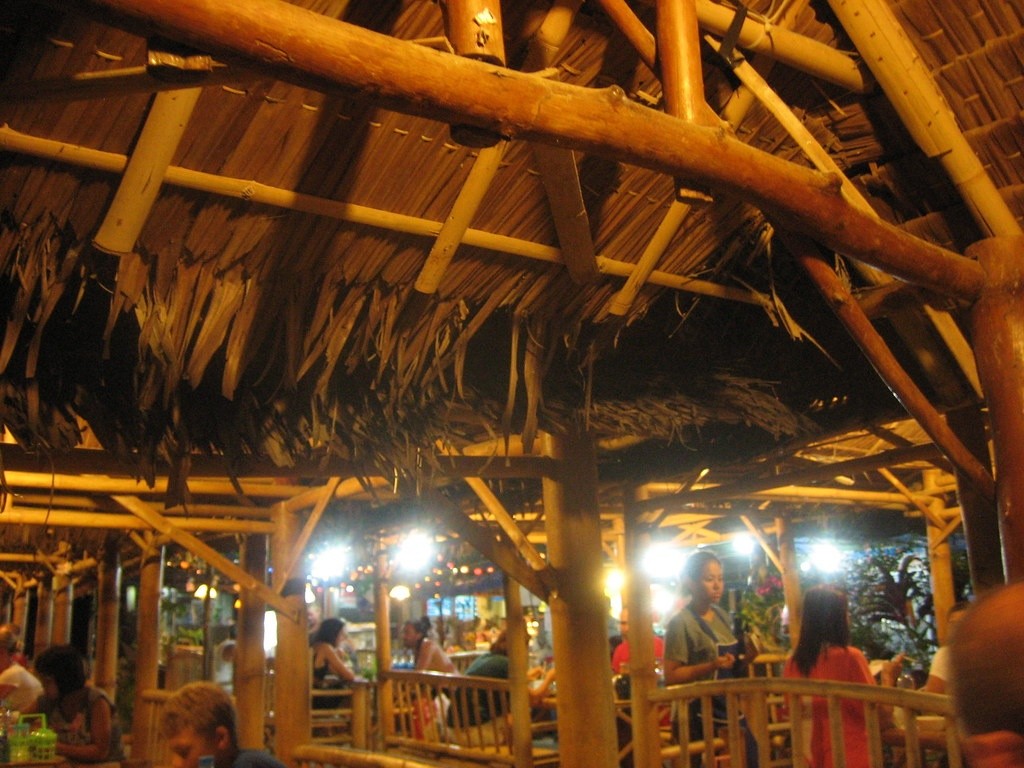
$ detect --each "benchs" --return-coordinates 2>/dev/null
[480,742,559,768]
[310,680,367,750]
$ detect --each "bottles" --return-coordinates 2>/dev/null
[391,654,415,669]
[612,662,631,700]
[364,654,374,678]
[897,667,915,689]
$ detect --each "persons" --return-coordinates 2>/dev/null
[946,581,1024,768]
[664,552,759,768]
[446,627,556,746]
[783,588,905,768]
[158,683,286,768]
[611,608,670,728]
[0,624,43,713]
[924,601,970,694]
[308,606,361,709]
[14,646,126,768]
[402,616,463,701]
[213,620,238,691]
